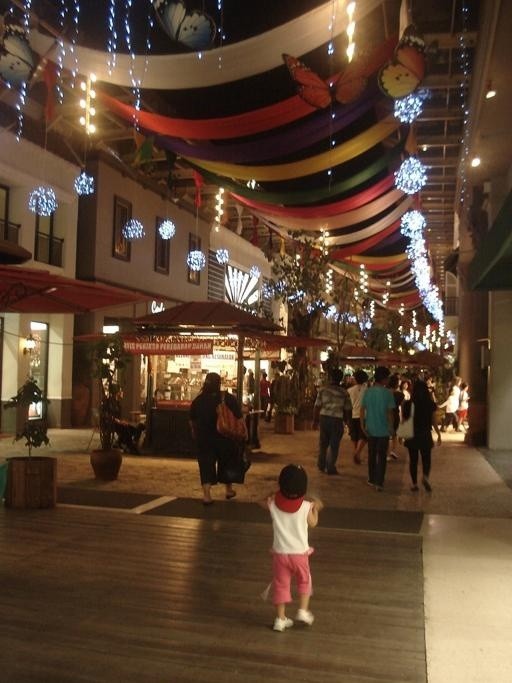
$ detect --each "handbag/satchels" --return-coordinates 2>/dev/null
[396,416,414,439]
[217,404,249,442]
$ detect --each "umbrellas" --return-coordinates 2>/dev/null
[1,264,159,315]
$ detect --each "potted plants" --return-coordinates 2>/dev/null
[270,357,298,434]
[91,333,125,480]
[4,382,57,510]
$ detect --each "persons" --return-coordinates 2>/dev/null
[102,383,142,455]
[310,368,353,474]
[399,379,442,491]
[186,372,246,505]
[246,367,470,465]
[257,464,325,631]
[360,367,395,490]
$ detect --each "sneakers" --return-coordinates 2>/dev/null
[295,608,314,626]
[318,451,432,493]
[273,616,294,632]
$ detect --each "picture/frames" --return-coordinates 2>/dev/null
[112,193,133,263]
[188,231,200,286]
[155,215,170,275]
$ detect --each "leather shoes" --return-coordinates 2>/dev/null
[226,492,235,498]
[204,499,214,505]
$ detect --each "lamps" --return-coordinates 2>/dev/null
[121,218,146,240]
[28,118,58,217]
[406,242,444,322]
[394,159,426,194]
[393,94,422,124]
[250,265,259,279]
[187,195,206,272]
[25,332,36,354]
[74,71,96,196]
[400,210,426,241]
[217,248,229,263]
[158,187,176,241]
